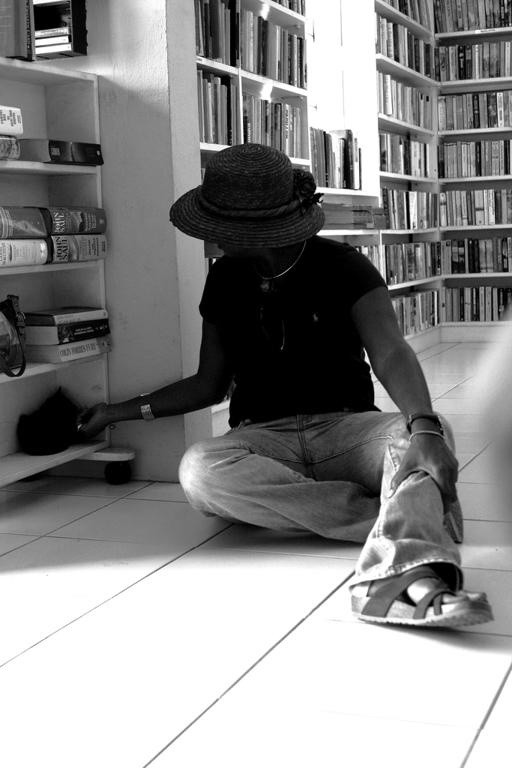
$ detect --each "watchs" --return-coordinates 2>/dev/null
[405,413,447,435]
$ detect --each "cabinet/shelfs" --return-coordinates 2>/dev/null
[435,0,511,324]
[374,0,441,337]
[194,0,314,412]
[1,59,110,489]
[315,184,383,377]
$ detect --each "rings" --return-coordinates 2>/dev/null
[77,424,82,432]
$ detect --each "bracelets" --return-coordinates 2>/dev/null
[409,430,446,441]
[140,392,156,421]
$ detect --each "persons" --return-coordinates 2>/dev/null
[77,143,494,630]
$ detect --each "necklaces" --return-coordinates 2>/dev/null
[256,239,307,293]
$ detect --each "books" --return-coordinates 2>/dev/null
[373,1,512,336]
[0,105,104,166]
[22,307,111,363]
[308,121,386,277]
[0,205,108,268]
[197,2,308,158]
[0,0,71,62]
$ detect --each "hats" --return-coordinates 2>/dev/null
[170,144,326,247]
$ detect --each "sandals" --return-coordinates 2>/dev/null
[350,571,496,626]
[446,502,463,543]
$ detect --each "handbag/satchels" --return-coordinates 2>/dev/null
[0,295,27,379]
